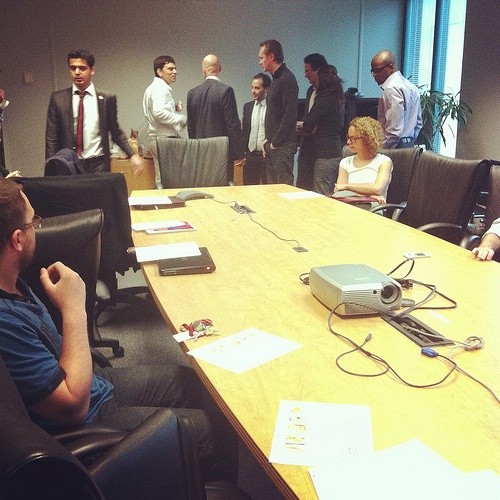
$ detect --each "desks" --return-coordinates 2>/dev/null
[130,183,500,500]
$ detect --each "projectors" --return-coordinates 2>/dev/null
[309,264,403,316]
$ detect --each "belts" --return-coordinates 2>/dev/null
[79,155,106,165]
[400,137,412,143]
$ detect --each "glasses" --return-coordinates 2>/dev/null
[21,216,44,231]
[371,65,389,74]
[345,136,362,143]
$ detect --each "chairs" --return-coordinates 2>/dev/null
[0,135,500,500]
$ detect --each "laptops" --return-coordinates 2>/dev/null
[133,195,184,209]
[157,246,216,276]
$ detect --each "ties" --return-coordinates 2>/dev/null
[75,91,87,154]
[248,105,263,152]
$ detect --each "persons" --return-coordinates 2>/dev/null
[241,73,272,185]
[0,176,281,500]
[258,39,299,185]
[471,217,500,261]
[45,48,144,176]
[187,54,248,186]
[143,56,186,138]
[296,53,345,195]
[331,116,394,216]
[370,51,423,149]
